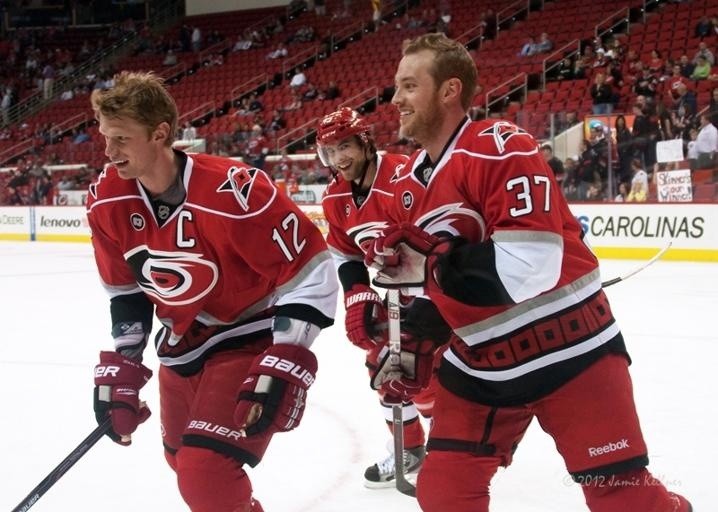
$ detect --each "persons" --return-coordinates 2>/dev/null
[80,68,337,511]
[363,32,693,512]
[314,107,457,491]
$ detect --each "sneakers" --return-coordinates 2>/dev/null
[365,447,426,489]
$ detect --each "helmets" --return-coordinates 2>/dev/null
[316,107,377,168]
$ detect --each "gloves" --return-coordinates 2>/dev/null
[342,285,455,409]
[93,351,153,446]
[358,222,464,288]
[233,343,318,439]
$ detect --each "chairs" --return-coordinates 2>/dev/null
[0,2,716,201]
